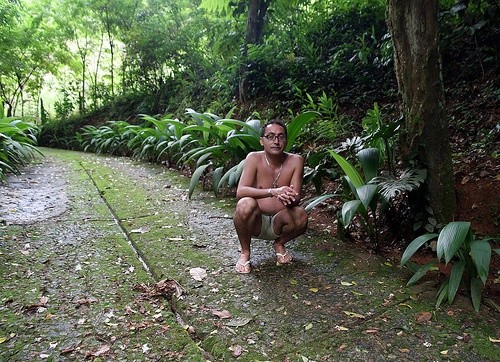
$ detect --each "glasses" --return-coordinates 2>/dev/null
[260,132,285,141]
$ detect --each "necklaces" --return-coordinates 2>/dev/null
[262,150,287,188]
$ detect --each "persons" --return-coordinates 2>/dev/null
[233,120,310,275]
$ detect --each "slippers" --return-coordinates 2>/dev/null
[271,245,292,265]
[235,260,251,275]
